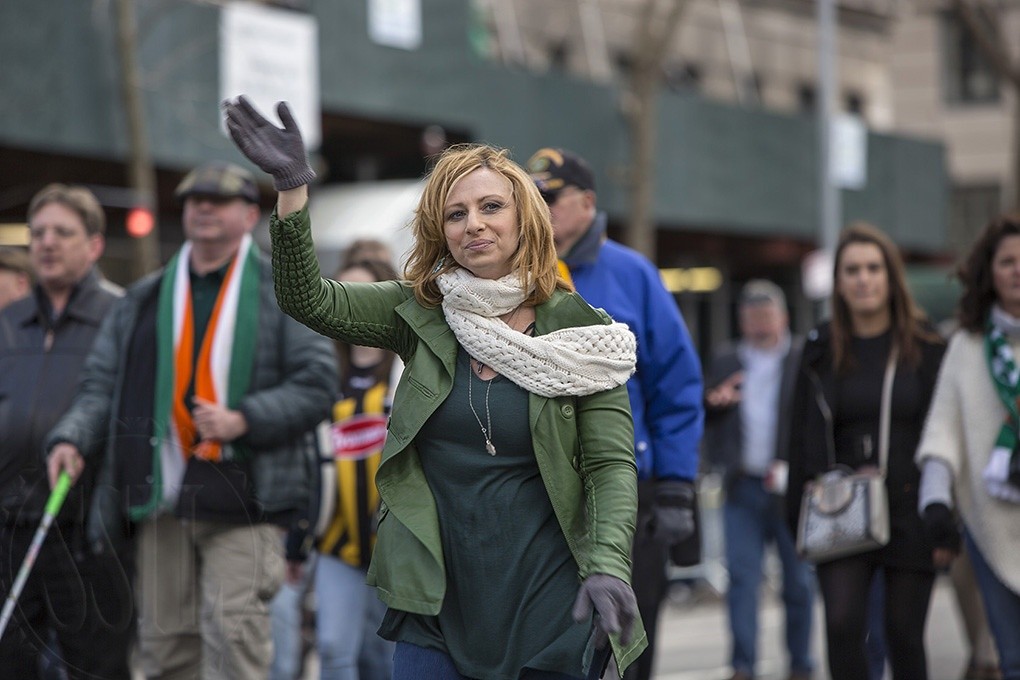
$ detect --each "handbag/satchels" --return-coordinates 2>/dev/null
[797,468,887,561]
[923,502,962,551]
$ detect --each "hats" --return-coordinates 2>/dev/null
[522,148,594,195]
[176,160,262,205]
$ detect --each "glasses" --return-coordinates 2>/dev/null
[539,184,577,204]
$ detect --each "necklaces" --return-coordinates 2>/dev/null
[466,352,495,456]
[478,307,516,375]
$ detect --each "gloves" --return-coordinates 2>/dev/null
[573,573,639,644]
[639,479,694,547]
[221,94,319,191]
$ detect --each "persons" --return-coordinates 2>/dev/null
[866,324,999,680]
[521,148,704,680]
[225,96,650,680]
[784,223,948,680]
[709,278,817,680]
[44,156,340,680]
[916,221,1020,680]
[265,239,408,680]
[0,184,129,680]
